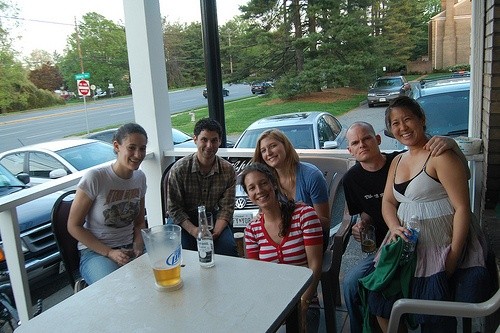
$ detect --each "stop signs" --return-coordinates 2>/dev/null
[77,80,90,96]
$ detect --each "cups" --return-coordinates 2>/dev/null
[206,212,214,230]
[360,224,377,255]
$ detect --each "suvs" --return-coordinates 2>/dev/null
[400,73,470,138]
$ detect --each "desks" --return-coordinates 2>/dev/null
[13,247,313,333]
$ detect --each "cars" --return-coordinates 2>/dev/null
[228,111,350,239]
[0,162,74,296]
[80,128,235,149]
[252,82,274,94]
[367,76,408,107]
[0,137,118,186]
[203,87,229,98]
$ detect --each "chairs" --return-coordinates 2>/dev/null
[52,157,500,333]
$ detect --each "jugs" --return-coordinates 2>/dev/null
[141,224,184,292]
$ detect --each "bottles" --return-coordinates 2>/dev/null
[196,205,216,268]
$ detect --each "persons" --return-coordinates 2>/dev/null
[366,96,487,333]
[67,122,147,285]
[240,162,324,333]
[343,122,471,333]
[162,119,238,257]
[255,128,330,333]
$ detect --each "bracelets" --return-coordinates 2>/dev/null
[299,296,310,304]
[107,249,111,256]
